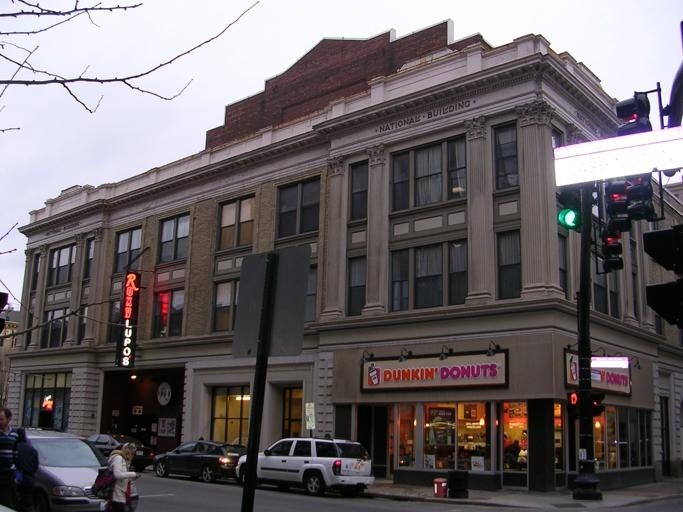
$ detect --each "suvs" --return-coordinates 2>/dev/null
[235,436,375,497]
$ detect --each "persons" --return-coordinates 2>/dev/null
[13,427,38,511]
[106,441,140,512]
[0,407,19,511]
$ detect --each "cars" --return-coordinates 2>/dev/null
[151,440,246,483]
[86,432,154,472]
[7,427,138,511]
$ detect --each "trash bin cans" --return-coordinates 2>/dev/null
[446,470,469,498]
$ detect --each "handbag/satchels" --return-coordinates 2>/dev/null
[90,467,115,503]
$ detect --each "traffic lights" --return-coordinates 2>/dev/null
[601,224,623,271]
[566,390,582,417]
[557,184,583,232]
[615,91,654,222]
[642,224,682,330]
[590,391,605,416]
[606,178,632,233]
